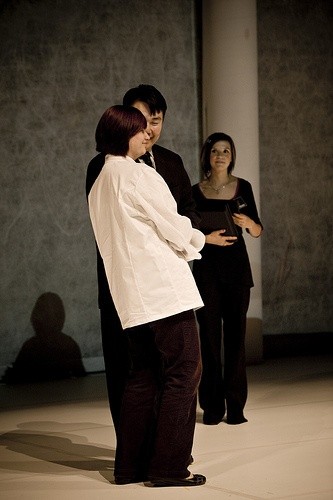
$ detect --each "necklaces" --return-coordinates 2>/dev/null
[206,177,234,194]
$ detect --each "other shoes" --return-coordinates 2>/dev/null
[114,474,206,487]
[202,412,249,426]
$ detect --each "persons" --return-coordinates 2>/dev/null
[192,132,264,424]
[86,83,193,465]
[86,104,205,488]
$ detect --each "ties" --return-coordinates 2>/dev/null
[139,151,155,170]
